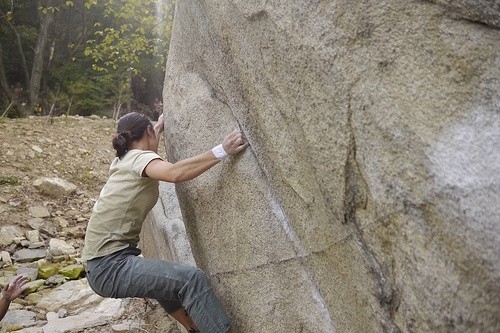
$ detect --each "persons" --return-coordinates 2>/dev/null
[0,275,29,322]
[80,111,250,333]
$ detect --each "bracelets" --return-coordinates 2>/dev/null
[212,144,228,161]
